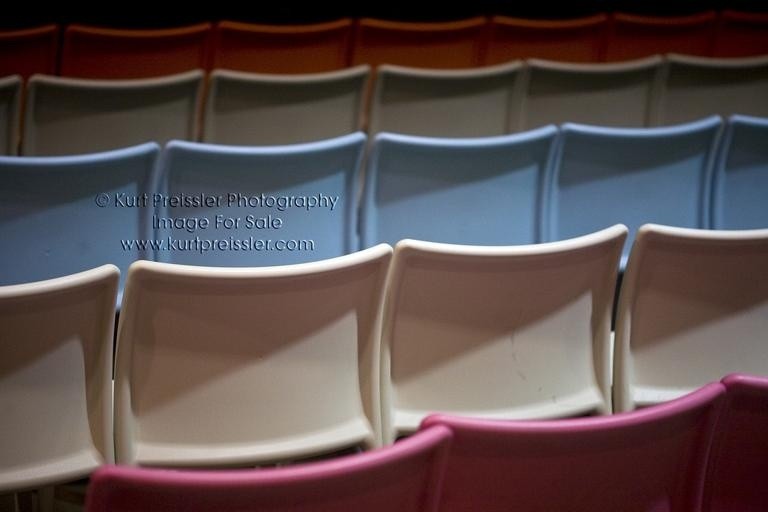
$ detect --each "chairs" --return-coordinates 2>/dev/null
[0,14,768,512]
